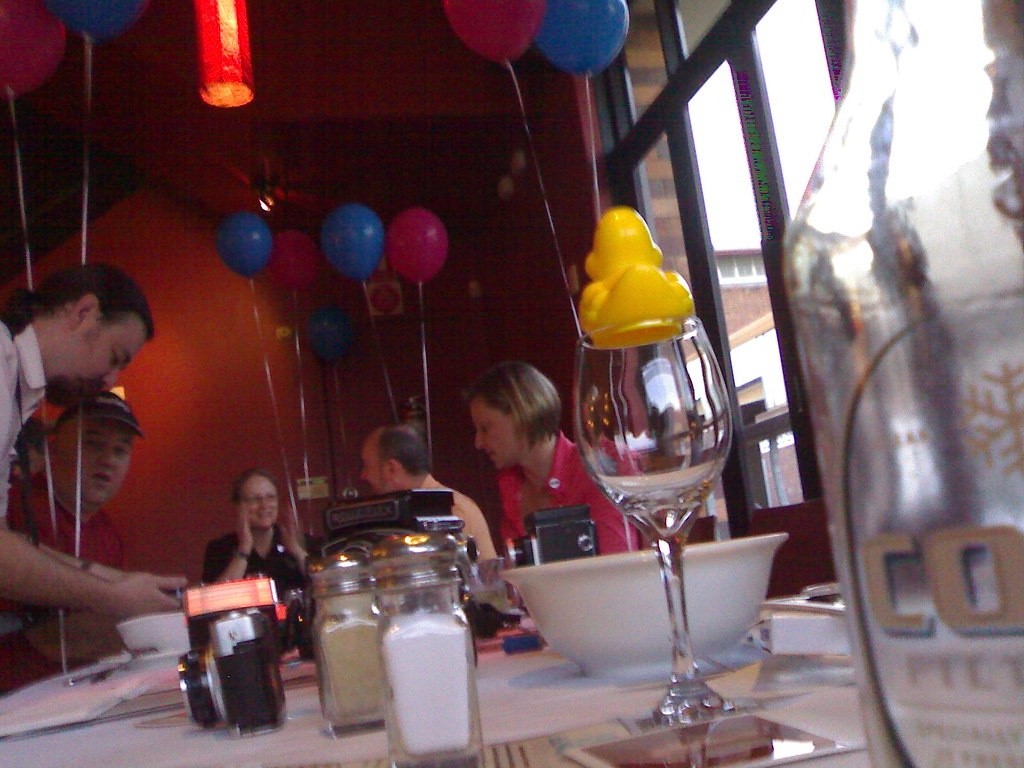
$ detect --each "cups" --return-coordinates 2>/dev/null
[114,611,190,657]
[461,557,510,612]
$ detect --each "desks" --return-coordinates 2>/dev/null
[1,612,875,767]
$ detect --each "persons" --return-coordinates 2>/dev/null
[0,259,187,621]
[466,361,651,567]
[203,467,315,653]
[0,390,144,693]
[357,424,497,564]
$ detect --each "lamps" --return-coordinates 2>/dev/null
[192,0,255,107]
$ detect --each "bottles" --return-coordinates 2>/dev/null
[372,532,484,768]
[779,0,1023,767]
[308,547,385,736]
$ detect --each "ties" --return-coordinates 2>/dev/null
[15,373,36,547]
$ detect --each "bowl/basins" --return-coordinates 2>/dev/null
[502,530,788,676]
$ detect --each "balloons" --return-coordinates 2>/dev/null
[262,230,320,298]
[215,212,272,278]
[441,0,545,65]
[307,302,353,363]
[321,204,384,282]
[47,0,149,42]
[0,0,67,100]
[532,0,631,76]
[387,208,450,285]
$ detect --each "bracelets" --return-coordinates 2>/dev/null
[233,551,250,561]
[296,551,309,560]
[81,560,91,571]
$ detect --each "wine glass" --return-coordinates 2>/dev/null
[570,318,743,733]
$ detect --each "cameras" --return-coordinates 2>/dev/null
[504,503,601,566]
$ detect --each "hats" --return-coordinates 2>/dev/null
[54,390,145,437]
